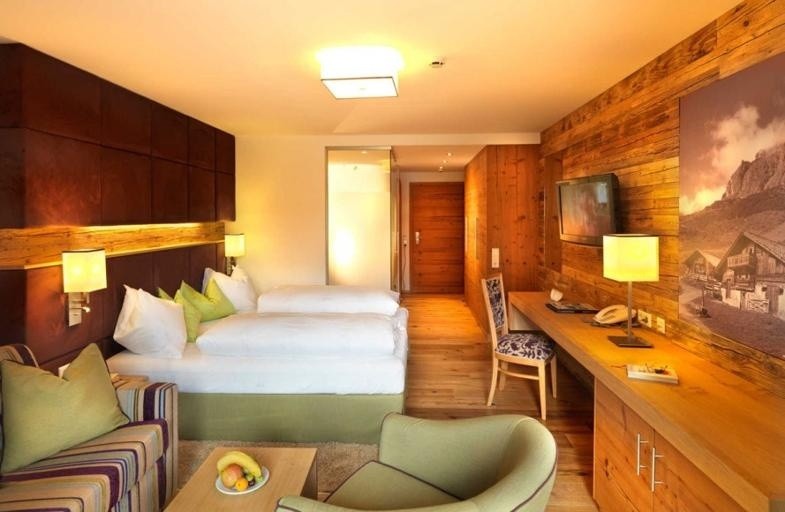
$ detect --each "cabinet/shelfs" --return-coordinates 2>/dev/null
[462,143,545,337]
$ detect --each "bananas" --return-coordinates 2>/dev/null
[217,450,262,477]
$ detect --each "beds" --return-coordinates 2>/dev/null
[257,282,403,315]
[103,307,408,446]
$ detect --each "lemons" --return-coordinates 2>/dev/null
[236,478,248,491]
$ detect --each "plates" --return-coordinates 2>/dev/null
[215,465,269,496]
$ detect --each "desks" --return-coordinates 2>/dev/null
[507,290,785,512]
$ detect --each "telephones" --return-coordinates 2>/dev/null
[592,304,637,326]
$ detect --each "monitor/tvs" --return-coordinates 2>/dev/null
[555,172,618,246]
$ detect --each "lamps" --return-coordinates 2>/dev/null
[602,233,661,348]
[224,234,246,275]
[316,47,402,103]
[62,247,109,326]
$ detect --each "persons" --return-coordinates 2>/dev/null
[575,185,609,237]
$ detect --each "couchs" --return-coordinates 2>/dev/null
[0,335,184,511]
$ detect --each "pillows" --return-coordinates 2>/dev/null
[114,265,258,357]
[4,341,134,469]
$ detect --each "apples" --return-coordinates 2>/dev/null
[221,464,243,488]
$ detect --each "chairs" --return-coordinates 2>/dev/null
[480,271,560,420]
[273,410,564,510]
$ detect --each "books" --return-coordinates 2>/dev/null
[625,363,680,385]
[545,300,600,314]
